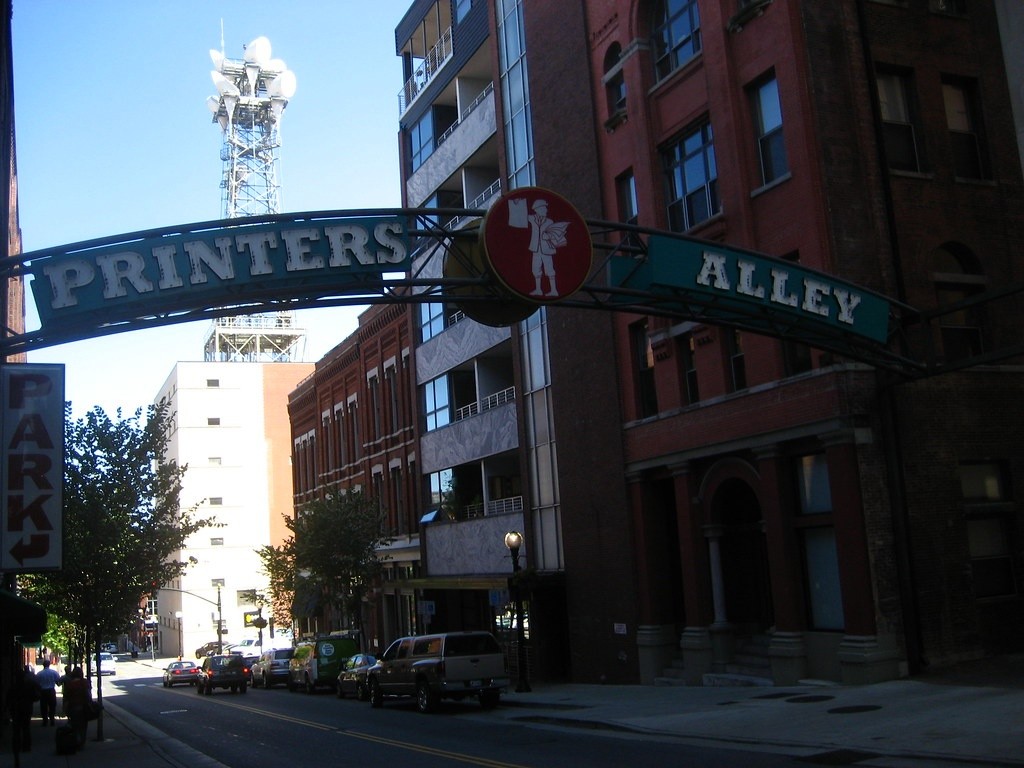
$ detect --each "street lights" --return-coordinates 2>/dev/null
[257,601,263,653]
[504,530,533,694]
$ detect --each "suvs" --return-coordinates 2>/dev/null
[247,646,294,689]
[196,652,250,695]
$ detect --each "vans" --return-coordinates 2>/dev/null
[288,637,358,693]
[229,638,285,657]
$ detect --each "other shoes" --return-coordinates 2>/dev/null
[50,719,55,725]
[41,721,48,726]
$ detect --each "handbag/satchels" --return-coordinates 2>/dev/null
[84,701,100,721]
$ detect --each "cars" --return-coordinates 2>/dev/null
[102,643,118,653]
[161,661,199,687]
[195,641,229,659]
[243,654,261,669]
[335,654,377,702]
[91,652,118,676]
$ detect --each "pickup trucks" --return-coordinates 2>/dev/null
[363,631,511,712]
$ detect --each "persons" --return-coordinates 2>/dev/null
[7,672,41,752]
[62,668,93,750]
[24,660,71,727]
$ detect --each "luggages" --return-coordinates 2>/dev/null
[57,725,77,757]
